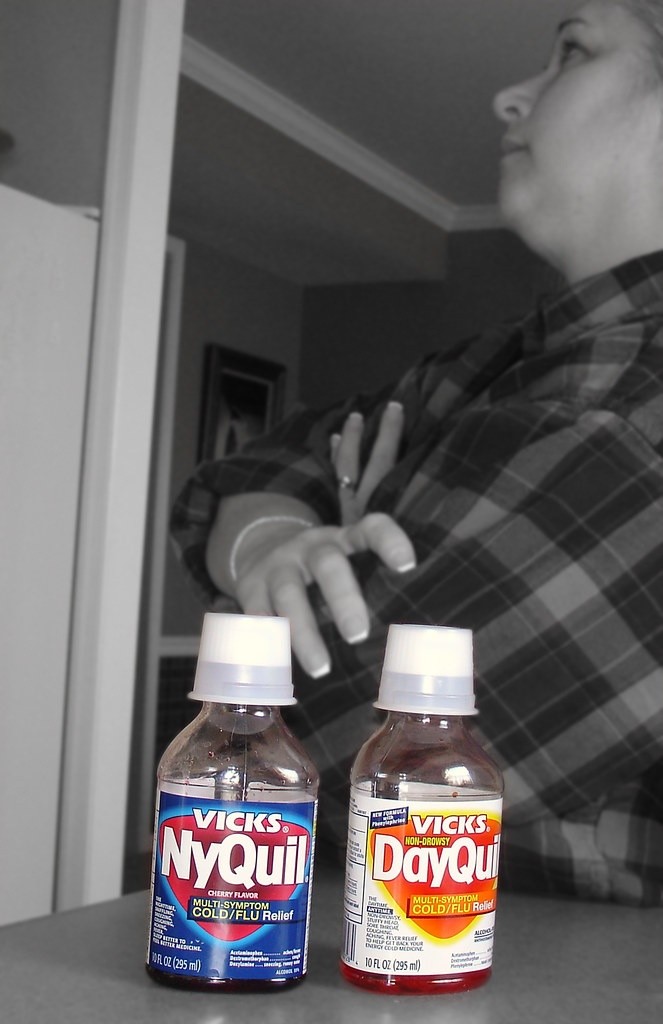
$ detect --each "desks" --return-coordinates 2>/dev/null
[0,861,663,1024]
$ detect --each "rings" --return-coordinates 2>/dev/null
[337,476,357,492]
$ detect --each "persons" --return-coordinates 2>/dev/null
[167,0,663,907]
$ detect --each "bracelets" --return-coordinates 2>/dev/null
[228,515,314,581]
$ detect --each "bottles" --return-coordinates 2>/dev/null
[144,613,320,992]
[340,624,505,995]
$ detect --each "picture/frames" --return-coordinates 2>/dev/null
[198,342,287,467]
